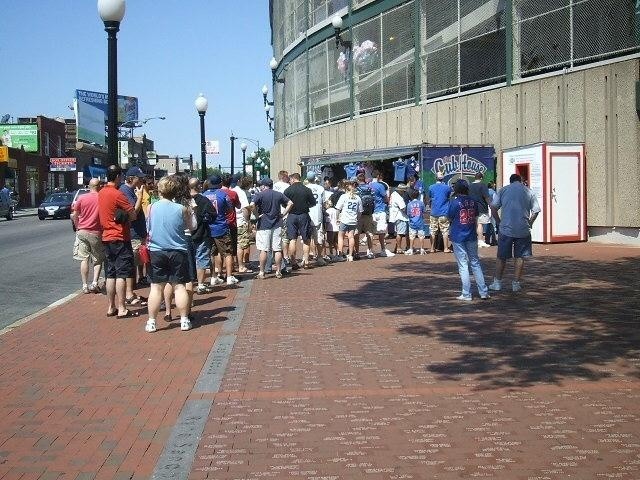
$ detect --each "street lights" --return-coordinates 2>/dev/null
[220,132,265,188]
[116,111,169,140]
[96,2,127,185]
[191,90,212,181]
[227,132,238,178]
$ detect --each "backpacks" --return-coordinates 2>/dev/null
[357,187,374,215]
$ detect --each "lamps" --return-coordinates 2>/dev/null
[262,84,274,132]
[270,57,286,92]
[331,11,352,53]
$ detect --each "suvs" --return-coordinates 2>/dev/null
[67,183,143,238]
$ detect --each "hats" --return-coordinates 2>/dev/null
[231,172,241,182]
[126,166,146,178]
[307,171,315,180]
[258,178,273,187]
[210,175,222,185]
[437,172,444,180]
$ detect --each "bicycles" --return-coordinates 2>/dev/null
[0,190,15,218]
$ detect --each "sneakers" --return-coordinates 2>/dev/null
[145,320,156,332]
[136,275,150,288]
[83,284,101,294]
[180,314,194,330]
[366,248,428,259]
[489,281,501,290]
[512,280,521,292]
[210,263,283,286]
[481,295,491,299]
[478,240,498,248]
[285,248,360,270]
[197,285,213,294]
[456,295,472,301]
[160,300,176,322]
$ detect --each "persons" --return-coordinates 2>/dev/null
[69,162,293,333]
[273,167,428,275]
[424,171,541,300]
[8,185,20,214]
[45,187,70,201]
[0,184,9,198]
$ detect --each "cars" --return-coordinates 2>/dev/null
[35,190,75,218]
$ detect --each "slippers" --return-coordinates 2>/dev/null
[430,249,439,252]
[444,249,453,253]
[107,293,148,319]
